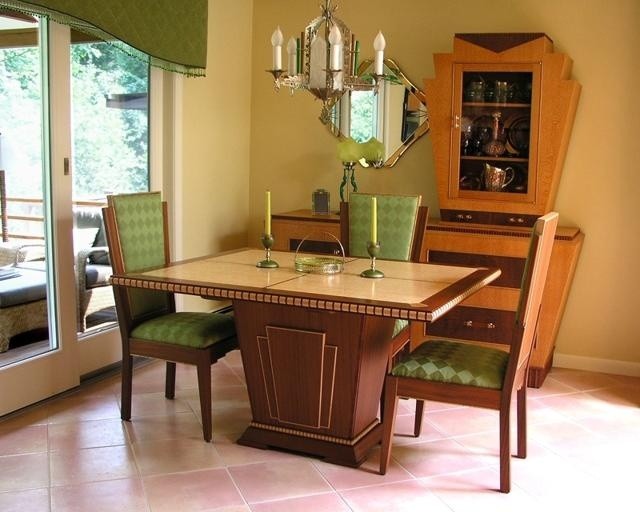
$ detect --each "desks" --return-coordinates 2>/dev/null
[110,246,502,468]
[270,210,585,389]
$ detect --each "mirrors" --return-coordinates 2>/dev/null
[318,56,429,168]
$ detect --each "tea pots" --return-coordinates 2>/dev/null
[483,162,514,191]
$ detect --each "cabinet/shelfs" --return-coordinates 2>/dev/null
[423,33,581,226]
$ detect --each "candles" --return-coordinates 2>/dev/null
[265,192,271,238]
[370,198,377,245]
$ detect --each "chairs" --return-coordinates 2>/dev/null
[340,192,429,338]
[0,206,116,353]
[102,191,238,442]
[380,211,558,494]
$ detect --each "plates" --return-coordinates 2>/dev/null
[504,112,529,156]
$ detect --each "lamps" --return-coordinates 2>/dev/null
[335,140,364,215]
[363,137,385,167]
[265,0,387,109]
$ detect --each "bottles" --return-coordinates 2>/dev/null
[471,80,486,101]
[496,79,510,105]
[484,113,505,157]
[464,125,479,156]
